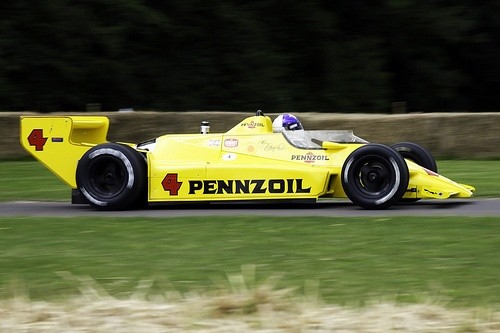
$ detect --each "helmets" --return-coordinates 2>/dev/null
[273,113,303,132]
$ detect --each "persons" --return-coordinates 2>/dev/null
[272,113,304,133]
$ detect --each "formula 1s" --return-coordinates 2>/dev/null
[17,115,476,210]
